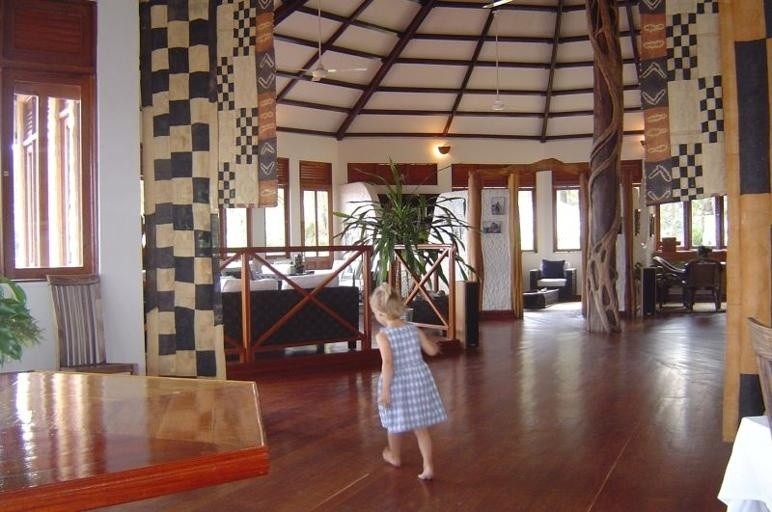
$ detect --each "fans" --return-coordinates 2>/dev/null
[279,0,368,83]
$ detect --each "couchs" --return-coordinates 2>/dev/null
[217,253,454,364]
[528,258,578,303]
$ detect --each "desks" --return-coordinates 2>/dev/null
[0,369,273,509]
[522,287,560,309]
[713,411,772,510]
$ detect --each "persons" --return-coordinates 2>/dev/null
[368,282,447,481]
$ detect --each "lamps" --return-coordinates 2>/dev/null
[490,8,507,113]
[436,144,451,156]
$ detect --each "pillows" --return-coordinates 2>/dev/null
[541,259,566,279]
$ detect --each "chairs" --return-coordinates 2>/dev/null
[745,313,772,434]
[44,271,142,377]
[643,254,727,318]
